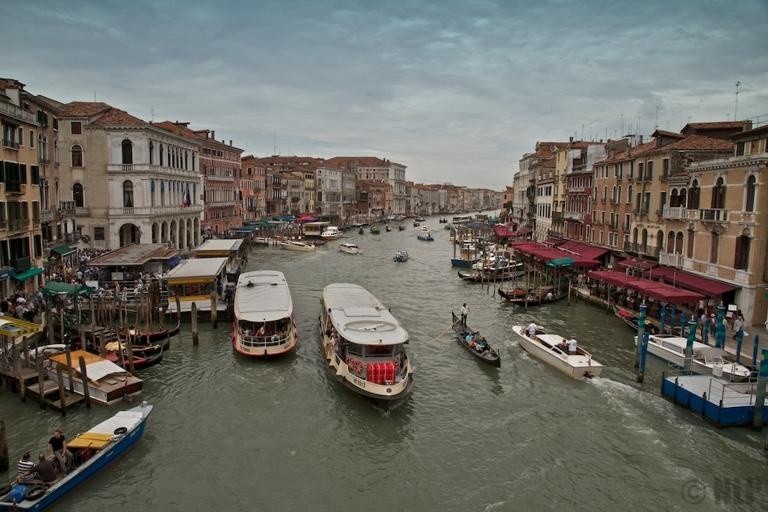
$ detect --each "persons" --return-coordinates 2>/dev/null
[546,291,552,301]
[465,330,485,352]
[322,312,345,363]
[16,454,36,477]
[559,337,577,354]
[528,319,537,338]
[241,323,289,345]
[48,431,71,476]
[36,455,55,484]
[461,302,468,324]
[264,220,307,243]
[587,274,746,341]
[3,249,164,320]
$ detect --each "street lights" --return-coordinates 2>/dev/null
[451,215,472,224]
[368,209,372,224]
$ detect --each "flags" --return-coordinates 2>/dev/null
[182,189,192,207]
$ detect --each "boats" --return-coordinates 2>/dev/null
[358,216,426,236]
[281,241,314,252]
[511,323,604,381]
[633,333,750,383]
[393,249,408,263]
[317,283,414,411]
[165,256,236,321]
[612,304,655,334]
[438,217,449,223]
[229,270,298,357]
[326,373,414,445]
[451,240,525,281]
[0,400,153,512]
[304,221,331,237]
[320,226,343,240]
[337,243,357,255]
[449,311,501,371]
[22,285,180,407]
[415,225,434,241]
[498,285,569,307]
[477,361,505,399]
[194,238,245,281]
